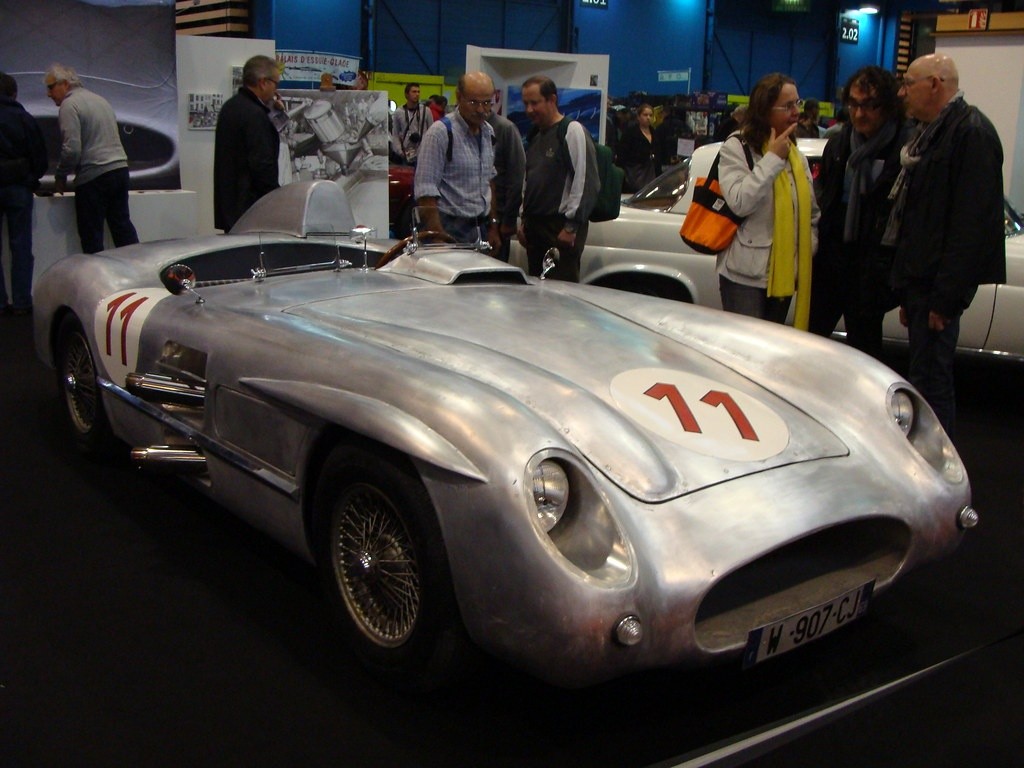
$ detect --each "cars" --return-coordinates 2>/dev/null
[505,138,1024,367]
[31,178,977,690]
[387,159,418,240]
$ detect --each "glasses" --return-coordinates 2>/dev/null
[900,76,945,86]
[264,78,280,88]
[771,99,803,112]
[461,92,492,105]
[845,96,881,111]
[46,81,58,90]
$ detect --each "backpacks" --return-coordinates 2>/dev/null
[556,117,625,223]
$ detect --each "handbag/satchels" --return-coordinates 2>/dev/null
[402,146,419,164]
[678,134,754,256]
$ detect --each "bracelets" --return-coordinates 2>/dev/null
[565,226,577,233]
[487,218,498,224]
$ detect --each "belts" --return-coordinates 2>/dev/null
[440,211,479,226]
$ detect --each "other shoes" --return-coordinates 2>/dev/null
[9,307,32,319]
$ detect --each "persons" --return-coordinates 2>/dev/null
[713,98,850,139]
[0,73,49,315]
[214,56,293,233]
[808,65,901,357]
[517,76,600,283]
[716,73,821,333]
[388,84,447,167]
[45,66,139,254]
[413,72,501,257]
[606,97,693,194]
[867,52,1006,445]
[487,110,526,263]
